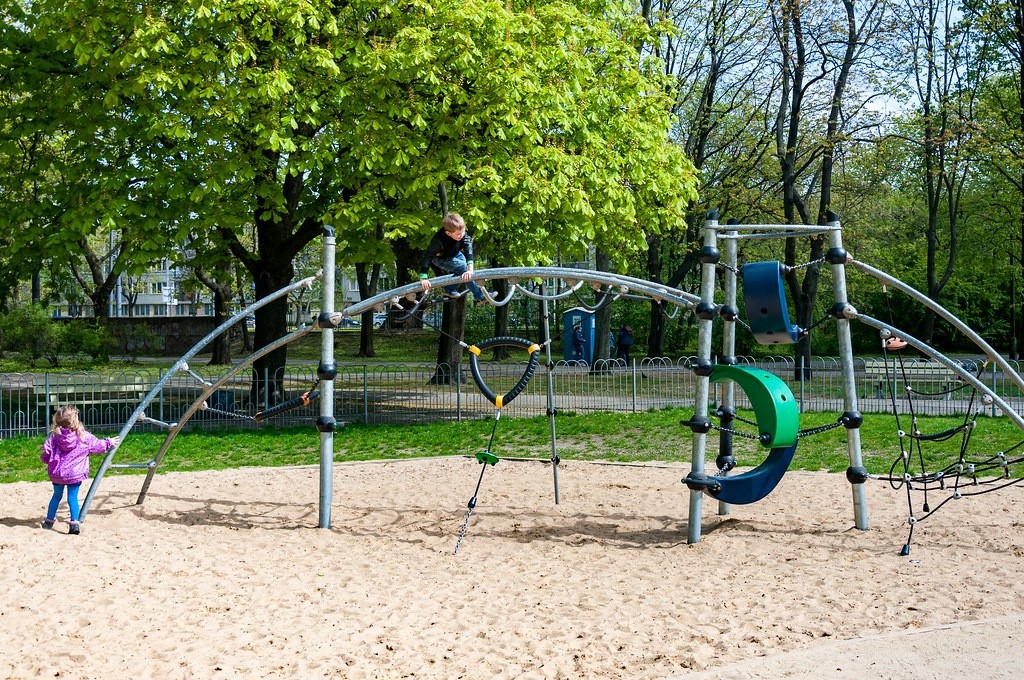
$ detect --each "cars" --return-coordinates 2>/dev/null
[422,313,443,327]
[338,319,361,328]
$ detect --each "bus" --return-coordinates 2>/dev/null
[246,316,255,328]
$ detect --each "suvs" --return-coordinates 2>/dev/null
[373,314,388,327]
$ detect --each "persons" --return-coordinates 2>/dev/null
[418,212,500,303]
[573,326,587,366]
[312,313,317,322]
[40,404,121,534]
[608,323,632,367]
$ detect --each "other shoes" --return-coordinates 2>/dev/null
[442,291,460,299]
[68,524,80,534]
[43,522,53,529]
[481,292,498,303]
